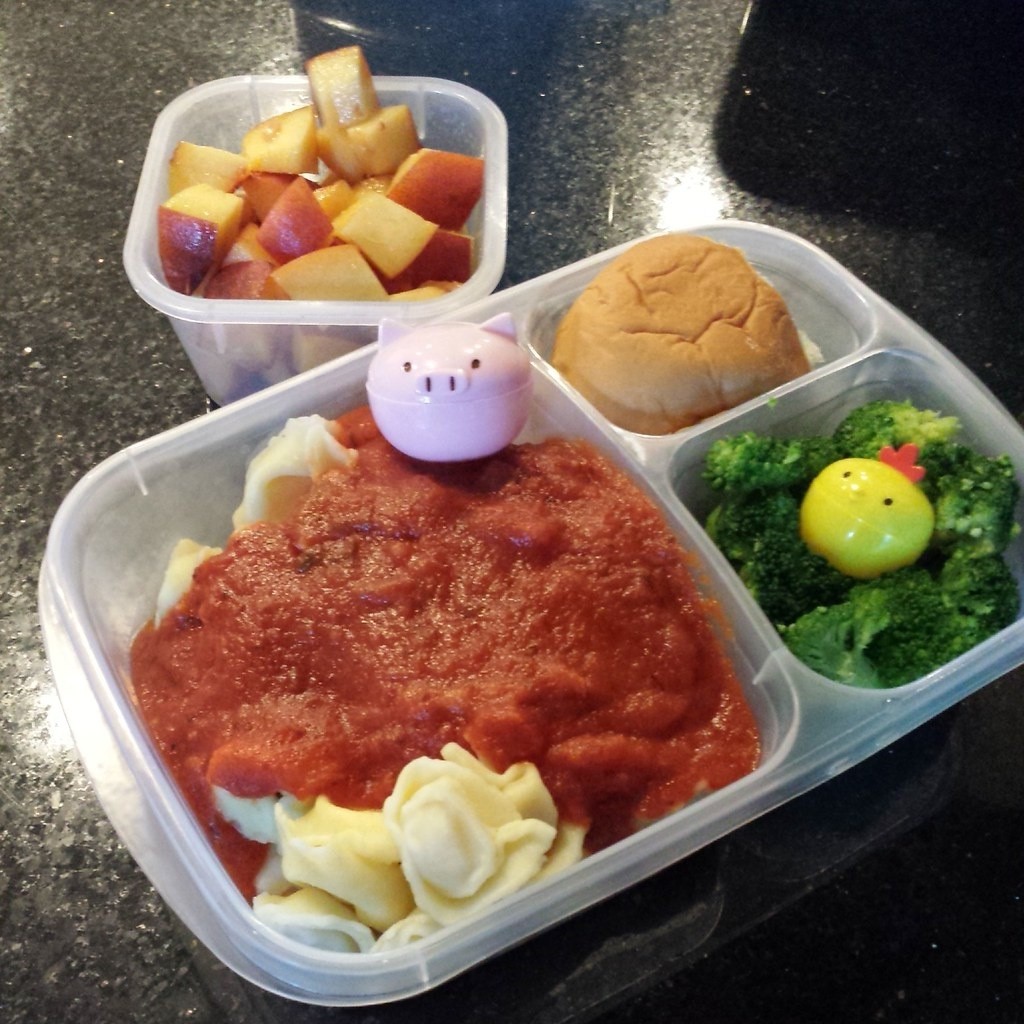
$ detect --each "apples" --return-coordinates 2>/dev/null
[156,44,485,399]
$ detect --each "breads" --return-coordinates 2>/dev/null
[546,234,811,437]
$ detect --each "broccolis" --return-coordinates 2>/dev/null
[695,403,1024,688]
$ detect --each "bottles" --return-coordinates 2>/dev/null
[711,0,904,110]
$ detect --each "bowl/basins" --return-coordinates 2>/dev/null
[37,74,1024,1007]
